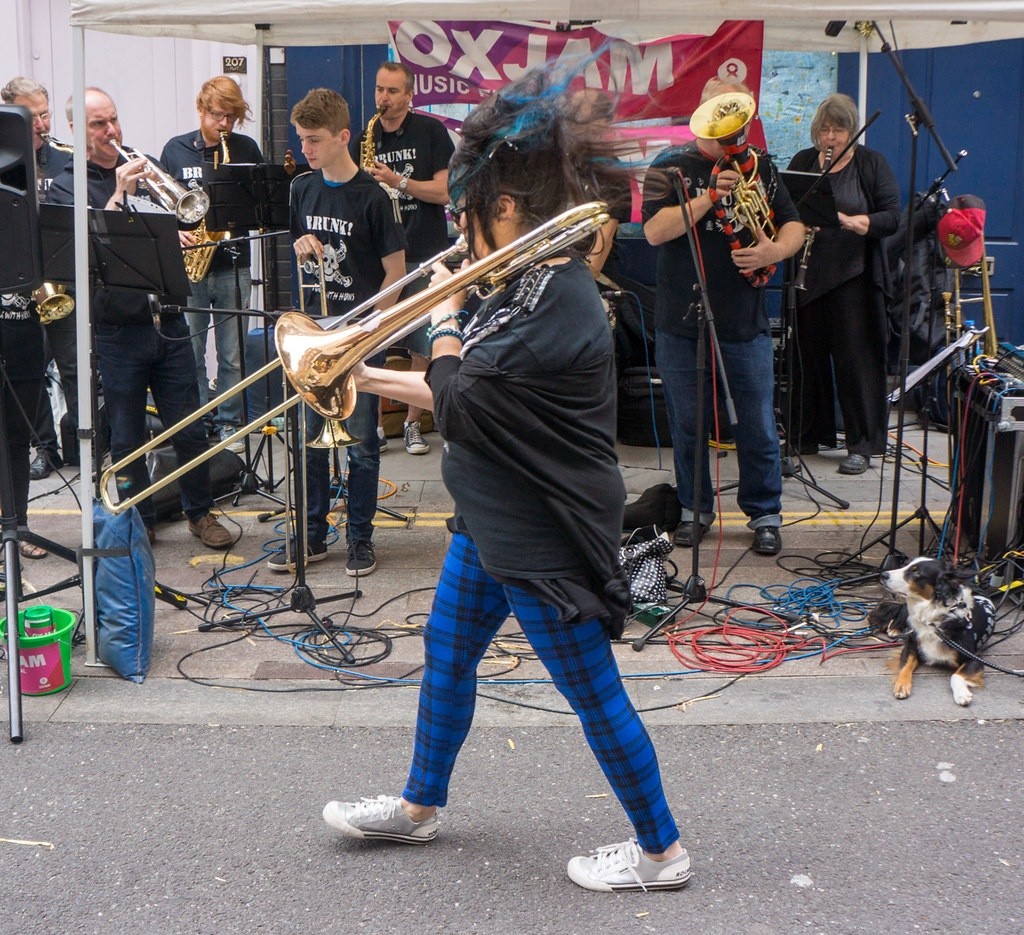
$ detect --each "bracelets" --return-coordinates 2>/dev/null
[426,309,469,336]
[429,327,464,346]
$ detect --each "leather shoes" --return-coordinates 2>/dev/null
[753,526,781,556]
[672,521,709,547]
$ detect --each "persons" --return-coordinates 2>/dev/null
[0,62,987,577]
[321,34,692,893]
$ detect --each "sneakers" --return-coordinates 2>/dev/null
[219,425,245,452]
[29,451,63,480]
[404,422,430,454]
[323,795,440,844]
[267,536,328,570]
[376,427,389,452]
[568,838,692,892]
[345,540,377,577]
[189,512,233,546]
[146,528,156,546]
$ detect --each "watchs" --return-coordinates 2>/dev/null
[397,178,408,192]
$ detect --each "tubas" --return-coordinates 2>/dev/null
[688,91,781,287]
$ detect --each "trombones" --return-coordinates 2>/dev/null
[282,235,338,576]
[104,201,614,516]
[941,217,1002,453]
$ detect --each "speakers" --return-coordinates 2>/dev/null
[1,104,40,299]
[711,325,789,444]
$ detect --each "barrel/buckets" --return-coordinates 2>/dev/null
[0,608,77,696]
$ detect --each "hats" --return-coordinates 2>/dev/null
[938,209,985,266]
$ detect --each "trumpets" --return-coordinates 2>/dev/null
[111,134,209,226]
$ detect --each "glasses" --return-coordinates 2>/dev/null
[206,108,239,122]
[30,109,53,125]
[448,203,476,228]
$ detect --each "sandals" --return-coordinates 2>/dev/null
[18,539,48,559]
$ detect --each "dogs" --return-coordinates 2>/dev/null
[868,555,997,705]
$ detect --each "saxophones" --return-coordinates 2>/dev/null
[183,128,236,284]
[20,140,89,323]
[354,105,398,169]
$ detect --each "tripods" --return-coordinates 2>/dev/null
[827,26,957,590]
[184,161,408,666]
[632,172,798,653]
[711,111,881,507]
[0,298,189,744]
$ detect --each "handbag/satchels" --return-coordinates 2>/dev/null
[618,525,678,604]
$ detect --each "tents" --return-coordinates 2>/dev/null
[69,3,1024,663]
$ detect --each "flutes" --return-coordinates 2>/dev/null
[792,143,836,299]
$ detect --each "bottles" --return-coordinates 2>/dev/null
[271,417,291,431]
[24,605,53,637]
[959,320,980,365]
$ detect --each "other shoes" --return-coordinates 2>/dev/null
[839,453,870,474]
[780,440,819,457]
